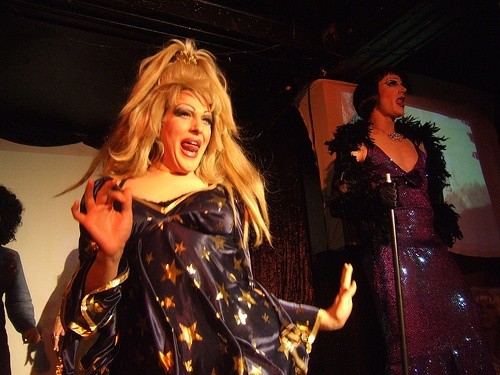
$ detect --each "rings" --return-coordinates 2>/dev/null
[53,335,59,340]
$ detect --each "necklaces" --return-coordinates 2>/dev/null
[371,124,403,143]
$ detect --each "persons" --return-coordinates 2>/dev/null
[49,260,86,356]
[327,64,500,375]
[0,184,42,375]
[50,35,360,375]
